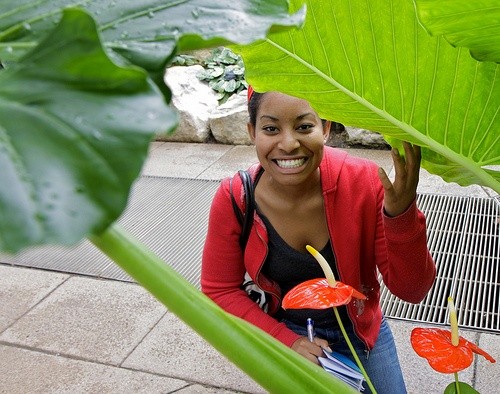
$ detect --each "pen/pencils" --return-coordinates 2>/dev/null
[307,317,318,345]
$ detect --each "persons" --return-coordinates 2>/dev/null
[199,83,437,394]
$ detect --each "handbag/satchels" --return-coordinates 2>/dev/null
[242,270,271,314]
